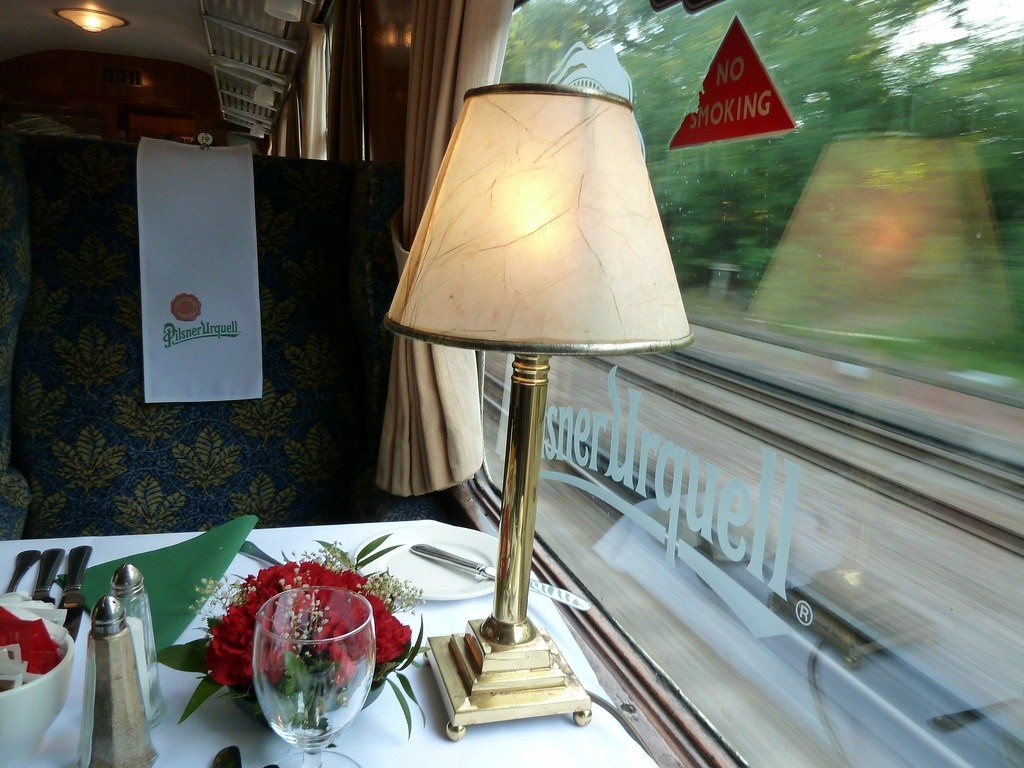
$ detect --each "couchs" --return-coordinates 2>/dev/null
[0,125,453,540]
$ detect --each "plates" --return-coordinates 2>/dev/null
[354,525,499,602]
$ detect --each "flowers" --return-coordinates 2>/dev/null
[154,533,432,741]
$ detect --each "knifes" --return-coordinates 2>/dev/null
[410,543,593,613]
[55,545,93,646]
[31,549,65,607]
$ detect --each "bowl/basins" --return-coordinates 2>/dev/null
[0,611,75,768]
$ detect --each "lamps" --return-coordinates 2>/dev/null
[745,127,1009,668]
[253,78,275,107]
[250,120,265,138]
[52,2,130,34]
[379,82,694,743]
[264,0,303,22]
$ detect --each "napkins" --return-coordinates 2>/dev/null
[52,514,258,655]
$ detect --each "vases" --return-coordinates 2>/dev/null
[229,675,388,734]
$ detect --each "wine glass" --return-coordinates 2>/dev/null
[249,583,377,768]
